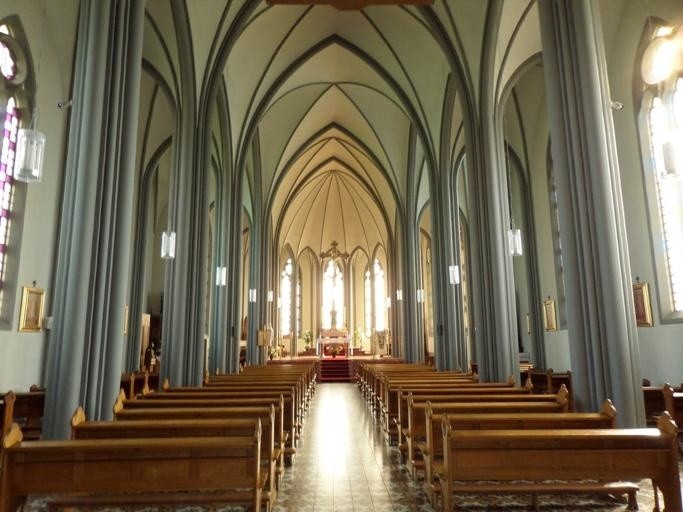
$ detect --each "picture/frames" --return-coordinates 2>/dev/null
[632,282,652,327]
[18,287,47,332]
[542,299,556,332]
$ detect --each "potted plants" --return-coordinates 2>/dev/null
[353,328,364,351]
[326,344,343,358]
[304,330,313,352]
